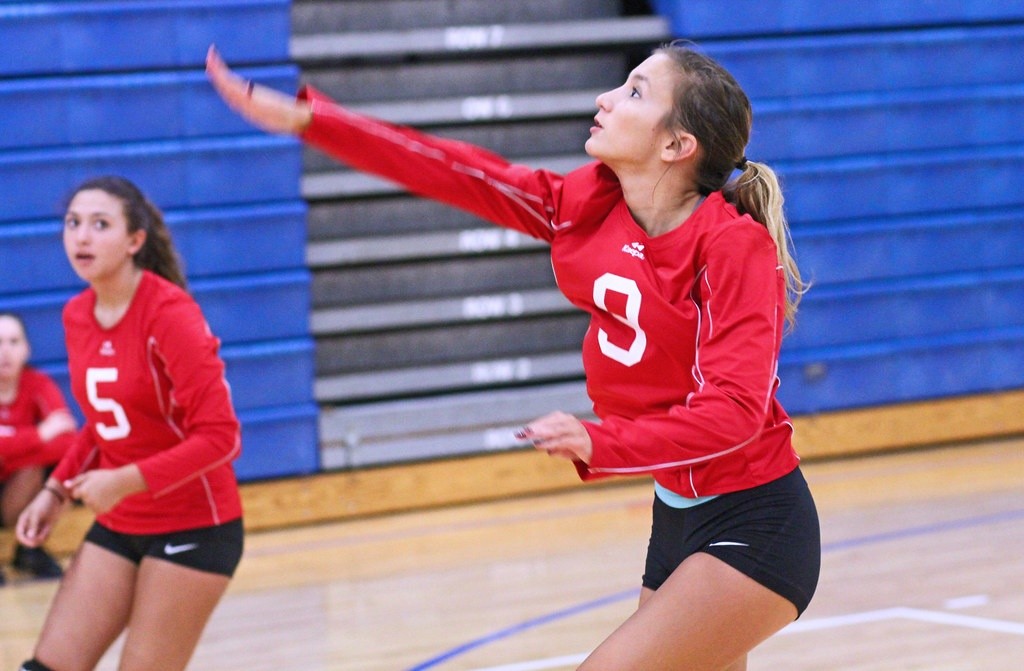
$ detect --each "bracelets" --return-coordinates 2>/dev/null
[43,484,67,506]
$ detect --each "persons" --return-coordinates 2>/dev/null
[15,177,244,671]
[205,34,820,671]
[0,311,78,586]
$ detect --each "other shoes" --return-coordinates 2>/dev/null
[10,541,63,580]
